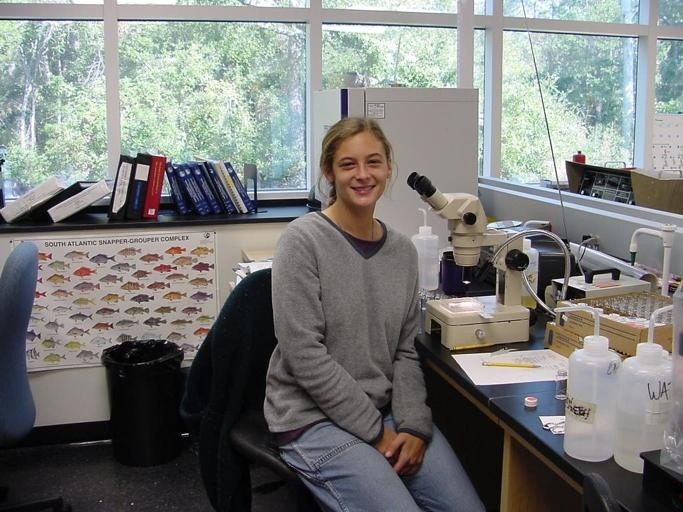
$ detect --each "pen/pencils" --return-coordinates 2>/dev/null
[449,343,495,350]
[482,362,541,368]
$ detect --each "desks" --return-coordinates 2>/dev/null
[413,299,683,512]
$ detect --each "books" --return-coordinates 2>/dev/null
[0,152,256,224]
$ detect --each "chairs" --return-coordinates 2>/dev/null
[0,243,65,512]
[181,267,299,512]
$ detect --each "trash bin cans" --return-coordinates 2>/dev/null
[100,340,184,467]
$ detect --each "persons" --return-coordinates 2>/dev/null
[261,116,491,512]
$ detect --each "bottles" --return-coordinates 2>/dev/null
[520,239,539,310]
[411,208,439,293]
[552,304,676,472]
[573,150,586,166]
